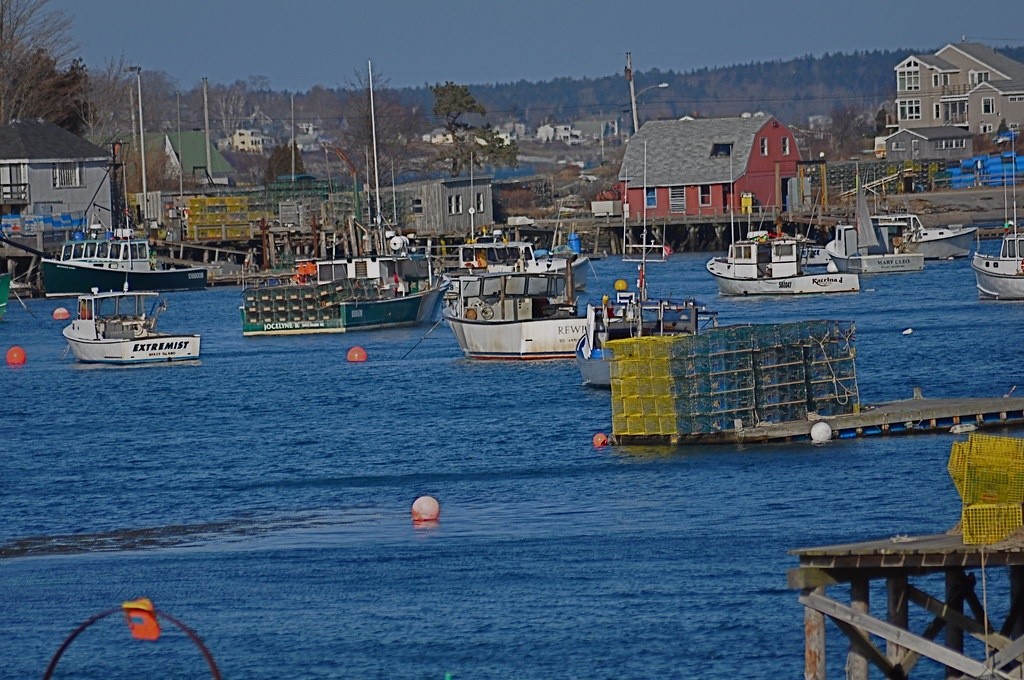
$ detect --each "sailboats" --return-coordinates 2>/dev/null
[440,151,590,303]
[574,138,719,391]
[970,128,1024,304]
[236,56,447,338]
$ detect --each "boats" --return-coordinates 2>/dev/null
[824,218,926,277]
[702,142,864,298]
[39,137,208,301]
[436,266,696,363]
[60,268,201,365]
[871,211,981,261]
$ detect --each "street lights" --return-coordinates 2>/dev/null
[121,65,150,239]
[624,49,670,132]
